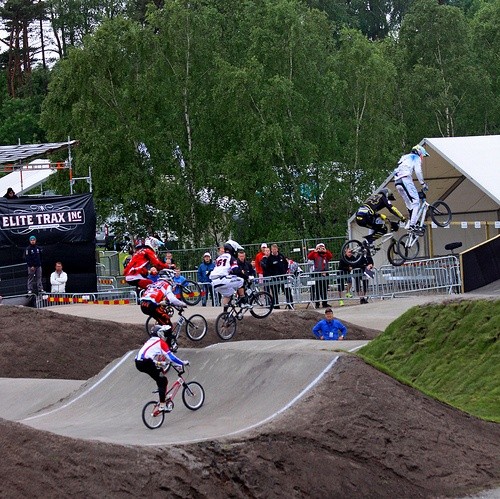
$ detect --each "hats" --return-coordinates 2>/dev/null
[30,236,36,240]
[203,253,210,257]
[261,243,268,248]
[325,309,332,313]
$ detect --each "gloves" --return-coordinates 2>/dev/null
[184,360,190,366]
[401,218,407,223]
[423,184,429,190]
[161,362,167,367]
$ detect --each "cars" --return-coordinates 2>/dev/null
[97,205,179,252]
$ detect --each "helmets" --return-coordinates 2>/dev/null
[224,240,245,258]
[149,325,172,341]
[378,188,396,202]
[145,236,165,255]
[159,268,177,285]
[411,144,430,160]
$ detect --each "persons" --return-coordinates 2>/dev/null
[5,188,16,199]
[311,309,348,341]
[49,262,68,298]
[133,324,191,412]
[24,235,46,295]
[196,239,303,309]
[106,225,192,352]
[305,243,333,309]
[392,145,430,232]
[337,247,374,306]
[355,188,407,248]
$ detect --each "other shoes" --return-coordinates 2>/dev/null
[39,289,45,293]
[171,344,177,351]
[274,306,281,309]
[28,290,32,294]
[313,293,368,309]
[288,305,293,309]
[363,241,370,249]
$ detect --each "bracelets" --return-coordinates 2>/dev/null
[341,335,344,338]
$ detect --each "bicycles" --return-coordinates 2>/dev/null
[146,268,201,338]
[397,185,452,259]
[341,217,408,266]
[215,280,274,339]
[153,302,207,373]
[142,362,205,430]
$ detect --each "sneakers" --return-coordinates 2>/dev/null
[407,224,421,232]
[159,404,172,411]
[152,388,159,393]
[241,303,253,309]
